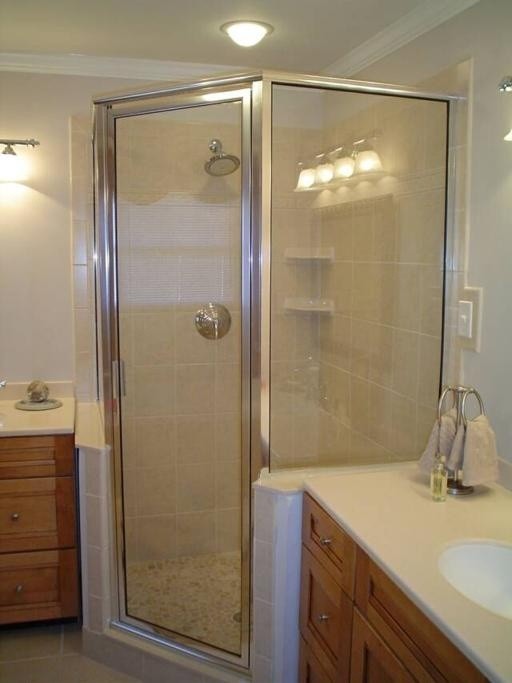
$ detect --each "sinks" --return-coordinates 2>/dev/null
[437,538,512,631]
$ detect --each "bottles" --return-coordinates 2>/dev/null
[429,463,448,503]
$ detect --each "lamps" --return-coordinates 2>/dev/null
[286,125,388,193]
[219,18,277,49]
[1,136,42,179]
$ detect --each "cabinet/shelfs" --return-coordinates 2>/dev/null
[1,429,82,629]
[280,244,337,320]
[295,486,359,682]
[348,530,493,683]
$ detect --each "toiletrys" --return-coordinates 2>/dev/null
[429,454,448,502]
[429,451,449,501]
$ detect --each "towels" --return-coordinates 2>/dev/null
[414,409,461,474]
[446,411,501,488]
